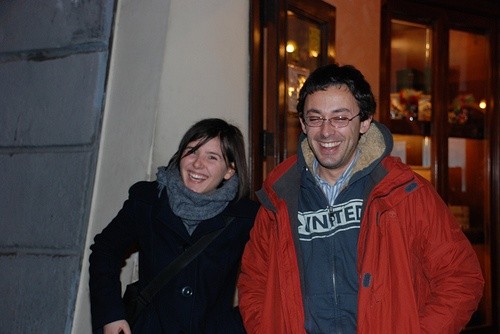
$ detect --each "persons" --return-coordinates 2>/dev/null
[237,64,485,334]
[90,119,261,334]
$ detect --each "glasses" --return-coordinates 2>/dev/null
[300,111,360,127]
